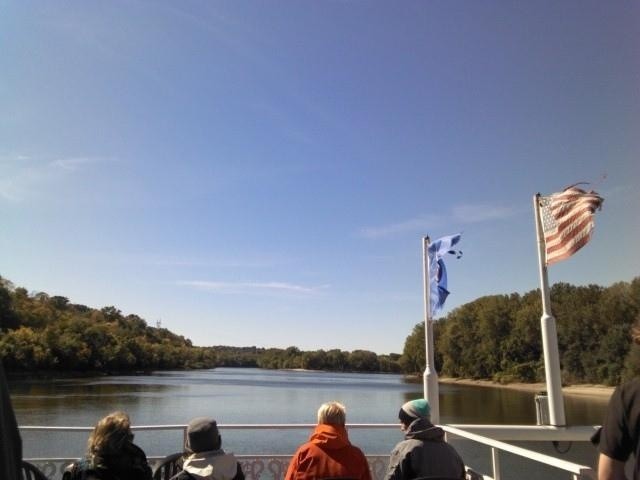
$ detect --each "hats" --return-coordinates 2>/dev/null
[184,416,222,454]
[398,399,431,426]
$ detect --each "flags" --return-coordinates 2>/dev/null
[426,230,464,319]
[537,180,606,267]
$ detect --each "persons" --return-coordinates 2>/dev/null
[383,398,466,480]
[168,417,247,480]
[596,376,640,480]
[71,409,153,480]
[284,400,373,480]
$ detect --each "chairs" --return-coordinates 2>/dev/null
[21,452,185,480]
